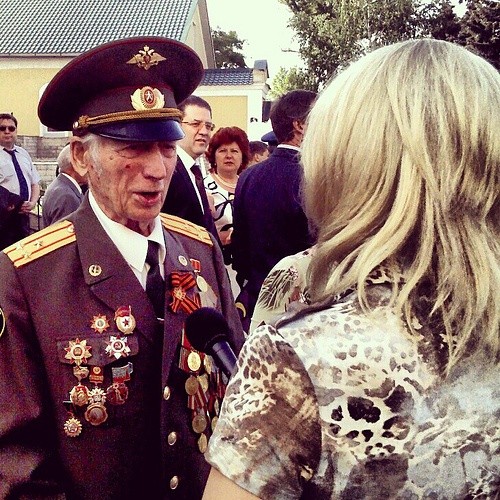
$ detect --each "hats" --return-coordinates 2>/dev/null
[261,131,278,153]
[38,36,204,141]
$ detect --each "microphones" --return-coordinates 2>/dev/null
[186,307,236,379]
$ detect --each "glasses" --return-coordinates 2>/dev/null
[182,121,215,131]
[0,126,16,132]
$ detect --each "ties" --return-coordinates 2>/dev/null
[3,148,29,201]
[145,240,166,320]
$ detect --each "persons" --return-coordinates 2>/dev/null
[231,90,319,318]
[201,39,500,500]
[160,95,230,284]
[203,126,252,301]
[42,143,88,228]
[239,141,270,176]
[0,36,246,500]
[0,113,40,251]
[261,131,278,158]
